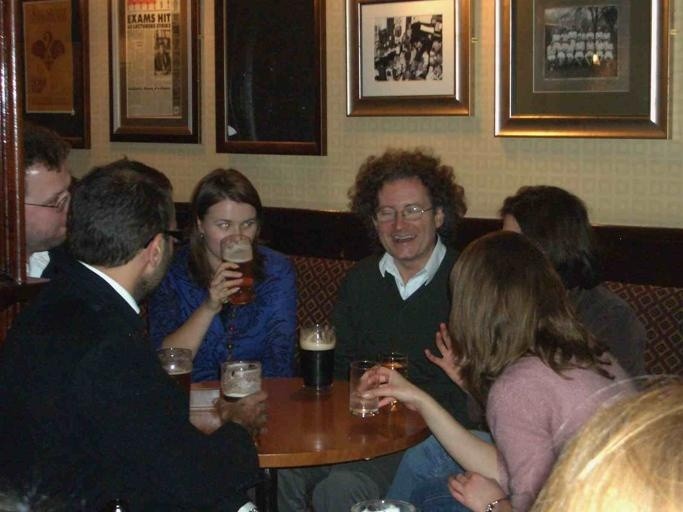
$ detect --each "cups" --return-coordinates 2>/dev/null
[378,350,409,413]
[299,324,336,392]
[219,360,263,443]
[153,346,192,411]
[350,361,381,417]
[219,234,256,305]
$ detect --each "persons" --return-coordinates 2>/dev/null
[531,373,683,512]
[425,184,645,398]
[277,147,481,512]
[357,232,641,512]
[546,25,615,71]
[148,168,298,385]
[23,119,73,279]
[0,153,271,512]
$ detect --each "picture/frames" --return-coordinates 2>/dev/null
[345,0,474,117]
[109,0,202,144]
[494,0,670,139]
[214,0,327,156]
[22,0,91,149]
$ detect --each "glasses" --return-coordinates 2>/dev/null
[144,224,191,249]
[373,204,436,224]
[25,195,69,213]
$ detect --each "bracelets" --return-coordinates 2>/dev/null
[486,496,510,512]
